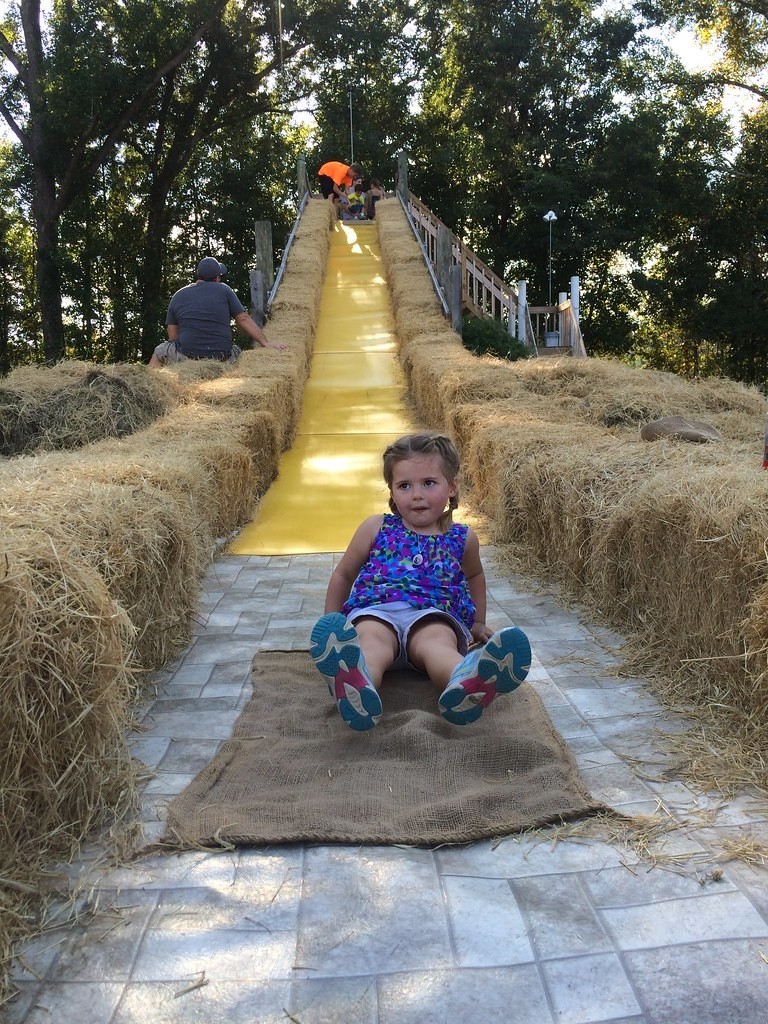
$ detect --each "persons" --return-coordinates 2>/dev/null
[318,160,386,219]
[148,257,288,369]
[309,435,532,731]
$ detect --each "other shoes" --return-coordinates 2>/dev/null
[359,216,365,220]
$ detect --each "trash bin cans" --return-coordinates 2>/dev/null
[542,330,560,347]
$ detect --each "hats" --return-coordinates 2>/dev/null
[198,257,227,278]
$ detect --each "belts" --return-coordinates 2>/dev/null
[182,352,231,362]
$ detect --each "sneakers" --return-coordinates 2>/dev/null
[310,612,383,731]
[437,627,532,725]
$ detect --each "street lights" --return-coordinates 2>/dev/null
[343,83,356,165]
[543,211,557,304]
